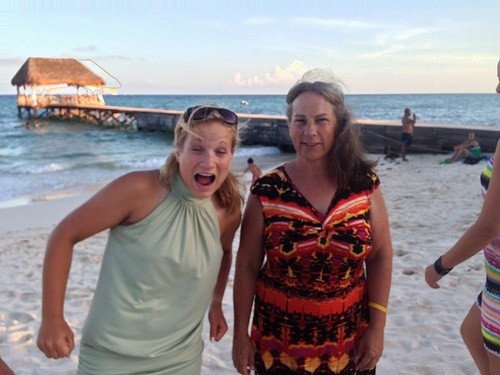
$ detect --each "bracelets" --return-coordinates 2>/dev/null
[368,302,387,313]
[434,257,453,275]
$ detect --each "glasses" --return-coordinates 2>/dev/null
[187,106,238,126]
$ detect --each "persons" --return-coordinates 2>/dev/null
[232,80,393,375]
[244,157,262,184]
[36,105,245,375]
[451,131,480,161]
[423,57,500,375]
[401,108,416,161]
